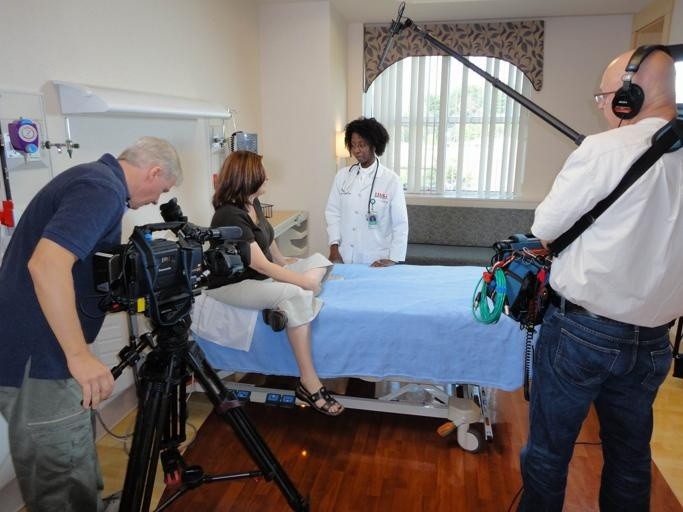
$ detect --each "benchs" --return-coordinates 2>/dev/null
[402,204,536,266]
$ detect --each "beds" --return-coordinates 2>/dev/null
[129,257,541,452]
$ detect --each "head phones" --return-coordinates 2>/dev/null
[612,44,672,119]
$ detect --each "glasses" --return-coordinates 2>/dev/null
[594,90,616,103]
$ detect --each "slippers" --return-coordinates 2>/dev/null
[262,307,287,330]
[295,382,344,415]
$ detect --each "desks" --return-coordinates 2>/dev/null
[263,208,307,240]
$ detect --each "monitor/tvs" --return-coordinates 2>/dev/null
[235,131,259,155]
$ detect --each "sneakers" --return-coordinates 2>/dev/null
[101,489,123,512]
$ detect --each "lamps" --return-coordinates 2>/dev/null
[336,131,351,158]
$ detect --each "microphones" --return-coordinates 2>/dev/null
[377,6,405,70]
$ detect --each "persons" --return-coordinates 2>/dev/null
[201,150,345,418]
[324,117,409,268]
[2,135,181,511]
[516,48,682,511]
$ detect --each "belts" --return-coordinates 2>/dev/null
[550,294,630,326]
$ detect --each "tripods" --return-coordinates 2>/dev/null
[118,322,311,512]
